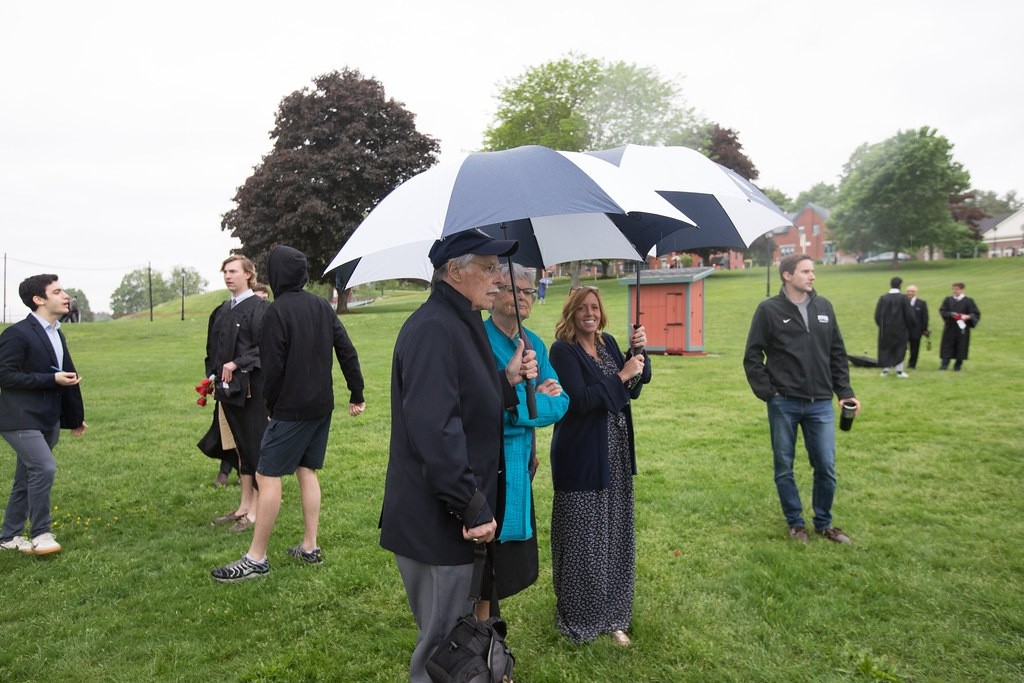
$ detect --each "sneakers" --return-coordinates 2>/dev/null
[30,532,61,554]
[285,544,324,566]
[211,554,270,583]
[0,535,33,553]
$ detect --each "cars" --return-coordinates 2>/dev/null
[709,257,726,267]
[855,250,913,265]
[987,245,1024,260]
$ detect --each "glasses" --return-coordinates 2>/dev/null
[469,259,503,277]
[498,284,537,295]
[568,285,598,297]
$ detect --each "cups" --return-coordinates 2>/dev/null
[839,401,856,431]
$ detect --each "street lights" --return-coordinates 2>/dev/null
[180,268,187,320]
[764,230,775,298]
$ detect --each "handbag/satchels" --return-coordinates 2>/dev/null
[426,614,515,683]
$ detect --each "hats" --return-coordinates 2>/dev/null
[428,227,520,269]
[891,277,902,288]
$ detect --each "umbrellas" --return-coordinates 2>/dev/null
[319,144,701,420]
[473,538,479,542]
[581,145,796,358]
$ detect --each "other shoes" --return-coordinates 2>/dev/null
[612,630,630,646]
[789,524,808,542]
[229,513,256,531]
[880,370,889,375]
[214,511,248,525]
[815,526,851,543]
[215,471,229,488]
[897,371,909,377]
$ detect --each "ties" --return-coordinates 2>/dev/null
[232,299,236,309]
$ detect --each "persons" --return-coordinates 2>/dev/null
[210,245,367,584]
[195,255,273,534]
[0,273,88,555]
[903,286,930,370]
[872,276,919,379]
[372,228,537,683]
[741,252,861,546]
[937,283,981,371]
[481,263,570,624]
[547,285,653,645]
